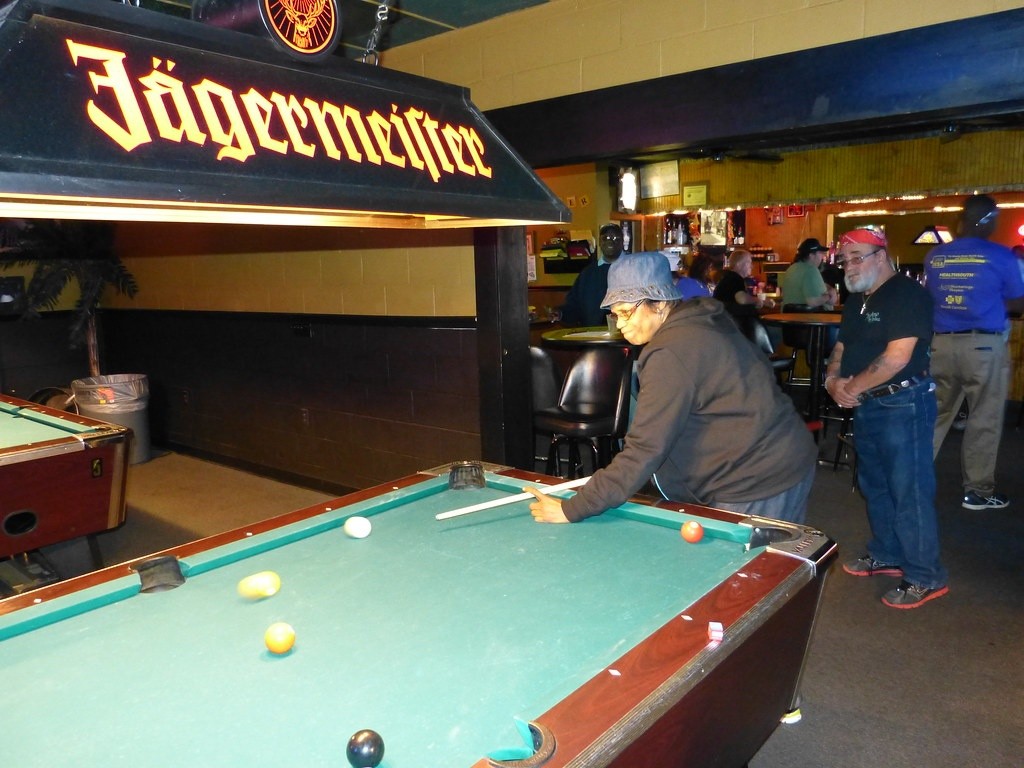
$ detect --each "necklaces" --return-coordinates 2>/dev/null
[859,272,894,315]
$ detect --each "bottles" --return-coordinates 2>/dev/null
[834,283,840,307]
[748,246,773,261]
[663,218,688,245]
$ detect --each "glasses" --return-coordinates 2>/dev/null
[608,298,646,324]
[835,249,883,269]
[601,235,621,243]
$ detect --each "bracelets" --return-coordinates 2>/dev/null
[825,376,837,394]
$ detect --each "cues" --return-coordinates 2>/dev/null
[435,475,592,521]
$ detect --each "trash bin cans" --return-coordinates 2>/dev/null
[71,373,149,465]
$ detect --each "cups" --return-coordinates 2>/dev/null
[606,314,624,339]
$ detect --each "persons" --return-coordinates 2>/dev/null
[712,249,762,344]
[674,255,713,302]
[523,252,820,724]
[778,237,840,415]
[827,229,950,609]
[1003,244,1024,320]
[922,194,1024,510]
[561,223,627,328]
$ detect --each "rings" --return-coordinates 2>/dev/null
[837,403,843,408]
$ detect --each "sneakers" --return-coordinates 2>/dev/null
[962,491,1009,510]
[881,579,948,610]
[843,553,903,577]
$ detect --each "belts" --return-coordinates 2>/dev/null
[936,330,997,335]
[855,370,929,402]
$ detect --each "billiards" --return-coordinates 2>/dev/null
[681,520,704,543]
[344,516,372,538]
[237,571,281,599]
[265,622,297,655]
[345,728,386,768]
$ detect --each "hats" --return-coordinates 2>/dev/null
[798,238,829,253]
[598,221,623,236]
[601,252,685,310]
